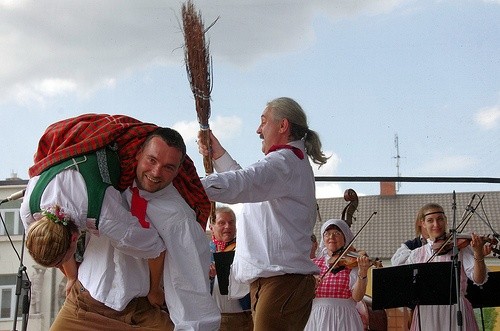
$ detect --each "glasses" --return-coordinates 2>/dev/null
[324,231,343,237]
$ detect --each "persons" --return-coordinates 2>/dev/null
[48,127,222,331]
[197,97,333,331]
[209,206,254,331]
[19,113,211,306]
[391,204,491,331]
[304,219,370,331]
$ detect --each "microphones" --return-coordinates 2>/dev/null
[462,194,476,219]
[3,188,27,203]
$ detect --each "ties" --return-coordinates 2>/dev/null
[265,144,305,160]
[129,186,150,228]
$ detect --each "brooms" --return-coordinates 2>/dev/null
[171,0,222,225]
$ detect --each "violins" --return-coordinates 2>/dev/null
[430,231,500,259]
[328,246,383,268]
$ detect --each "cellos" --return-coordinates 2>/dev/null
[341,188,387,331]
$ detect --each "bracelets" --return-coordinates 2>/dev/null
[474,257,484,264]
[358,275,367,279]
